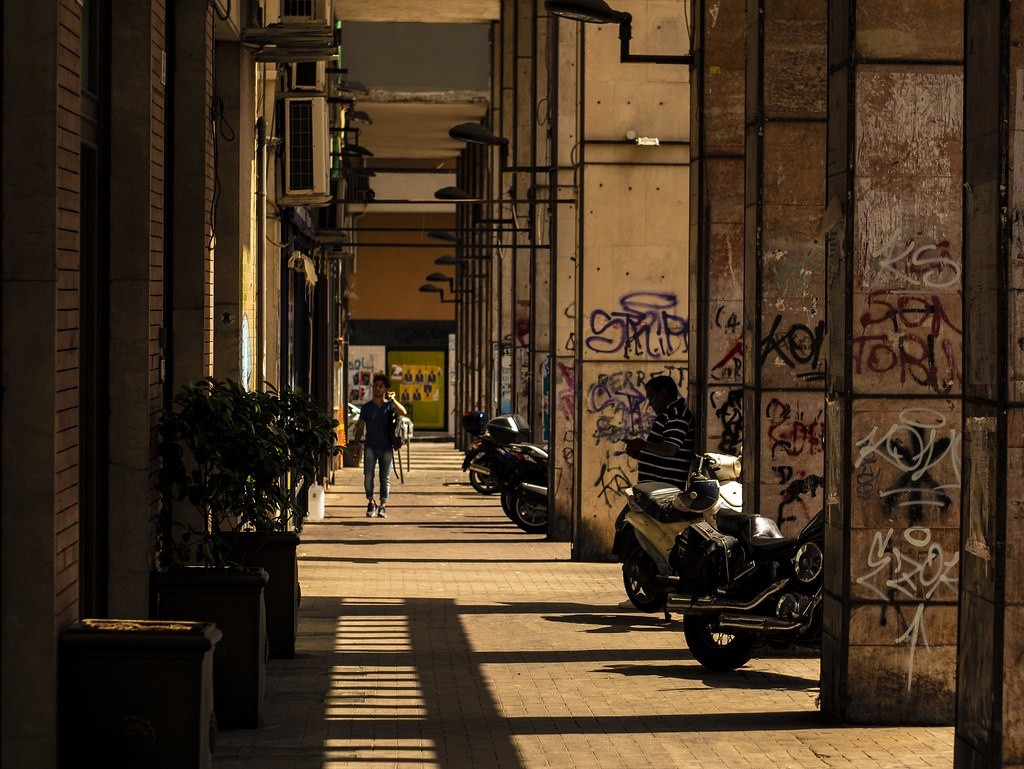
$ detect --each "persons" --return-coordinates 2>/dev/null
[619,376,696,609]
[355,375,408,518]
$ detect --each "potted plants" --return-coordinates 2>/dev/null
[150,385,267,732]
[193,377,355,660]
[343,439,362,468]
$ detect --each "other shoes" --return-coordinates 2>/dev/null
[619,599,636,608]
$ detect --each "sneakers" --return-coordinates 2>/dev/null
[366,499,378,517]
[378,506,385,517]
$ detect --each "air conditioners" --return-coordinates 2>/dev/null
[291,61,327,93]
[312,177,346,237]
[284,96,330,197]
[280,0,331,28]
[330,216,354,255]
[347,158,370,201]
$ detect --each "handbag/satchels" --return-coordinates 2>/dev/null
[386,401,405,451]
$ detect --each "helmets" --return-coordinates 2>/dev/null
[677,472,720,513]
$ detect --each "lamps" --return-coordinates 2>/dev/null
[340,80,370,96]
[448,121,532,173]
[543,0,692,66]
[348,110,373,125]
[434,186,513,225]
[426,272,471,293]
[434,254,486,279]
[419,285,460,303]
[427,231,492,260]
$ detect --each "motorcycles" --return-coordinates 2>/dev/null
[459,410,550,534]
[609,445,744,621]
[662,472,827,673]
